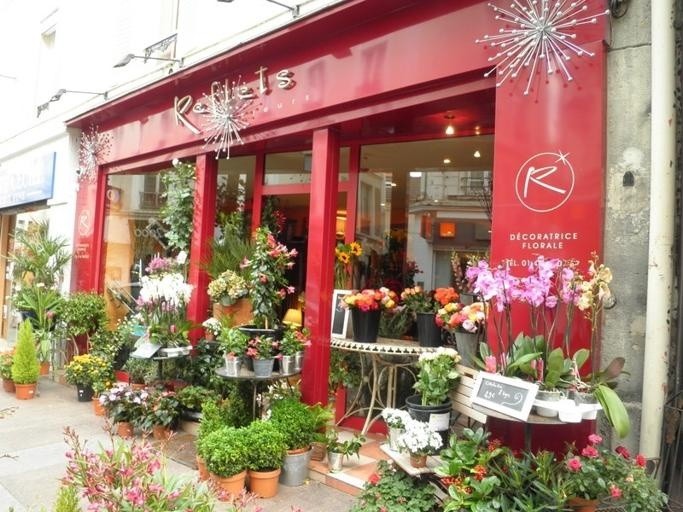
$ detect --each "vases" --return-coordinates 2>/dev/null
[349,309,381,343]
[150,424,173,443]
[154,342,194,357]
[415,311,443,349]
[114,422,136,440]
[565,496,600,511]
[280,353,303,375]
[223,356,241,377]
[217,293,237,308]
[73,380,96,402]
[252,356,274,377]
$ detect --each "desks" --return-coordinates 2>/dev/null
[470,405,567,458]
[328,337,457,441]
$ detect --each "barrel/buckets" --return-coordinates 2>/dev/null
[533,385,597,422]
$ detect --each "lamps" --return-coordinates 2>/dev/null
[35,99,58,117]
[443,112,455,136]
[50,88,109,101]
[111,54,182,71]
[474,136,481,158]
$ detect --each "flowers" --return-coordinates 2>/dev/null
[214,326,243,357]
[462,250,623,428]
[335,241,362,288]
[335,282,400,314]
[250,212,301,323]
[62,352,113,385]
[204,269,251,300]
[390,283,466,315]
[130,248,195,309]
[562,428,671,512]
[280,326,312,354]
[380,344,461,471]
[98,382,181,422]
[242,337,283,359]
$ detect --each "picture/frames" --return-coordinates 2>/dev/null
[329,289,354,340]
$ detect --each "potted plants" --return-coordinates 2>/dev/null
[56,285,106,353]
[0,212,74,401]
[92,373,111,416]
[323,426,367,472]
[196,389,328,500]
